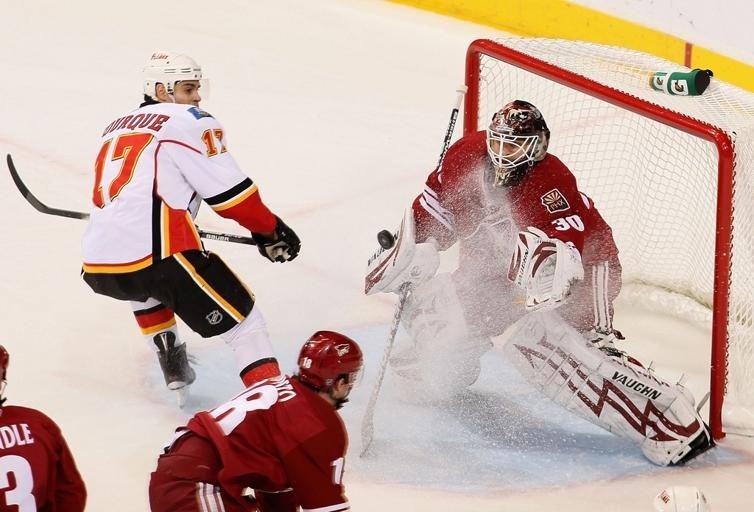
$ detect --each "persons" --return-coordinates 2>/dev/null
[0,346,88,512]
[149,330,362,509]
[80,50,301,392]
[363,99,716,468]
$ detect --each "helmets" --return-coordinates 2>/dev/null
[0,344,9,380]
[488,99,550,162]
[141,49,202,102]
[296,330,363,393]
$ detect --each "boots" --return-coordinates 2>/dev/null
[155,340,196,391]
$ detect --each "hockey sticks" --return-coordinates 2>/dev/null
[360,84,466,457]
[6,154,258,244]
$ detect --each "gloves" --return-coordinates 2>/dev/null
[506,226,584,311]
[363,207,441,296]
[250,214,302,264]
